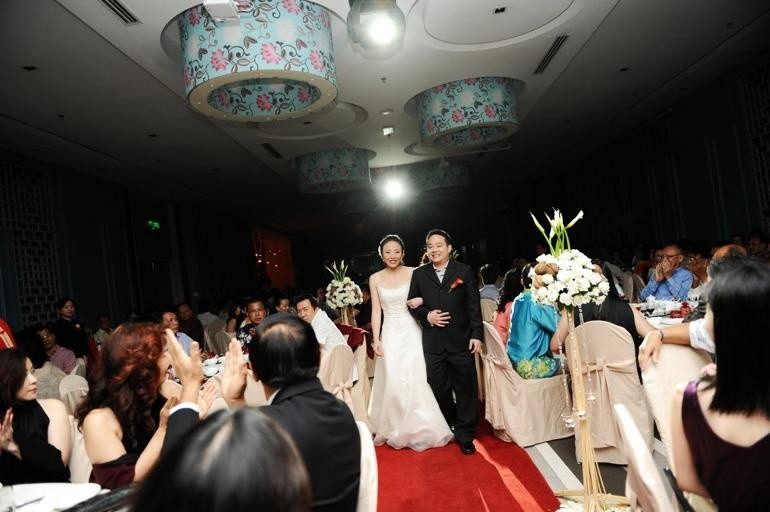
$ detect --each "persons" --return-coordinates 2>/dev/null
[0,284,372,512]
[672,256,770,512]
[479,237,770,381]
[367,230,485,455]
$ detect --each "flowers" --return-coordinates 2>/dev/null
[324,260,364,309]
[530,209,610,312]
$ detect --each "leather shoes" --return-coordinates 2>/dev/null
[456,439,475,455]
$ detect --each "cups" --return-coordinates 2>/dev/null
[0,486,15,512]
[633,295,693,318]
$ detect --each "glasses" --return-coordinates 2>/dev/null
[662,254,680,259]
